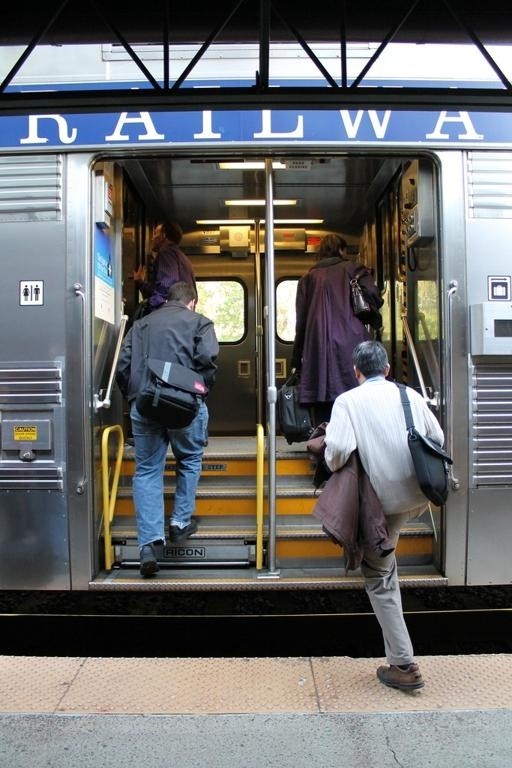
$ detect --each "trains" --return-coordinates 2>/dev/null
[0,43,508,592]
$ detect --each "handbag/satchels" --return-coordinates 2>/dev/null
[349,280,383,329]
[134,355,212,431]
[276,383,317,446]
[406,428,454,509]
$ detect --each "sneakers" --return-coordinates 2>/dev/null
[138,544,161,577]
[376,660,425,692]
[167,515,199,544]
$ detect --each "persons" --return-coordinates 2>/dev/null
[291,234,384,489]
[115,280,221,576]
[325,341,445,690]
[125,220,197,447]
